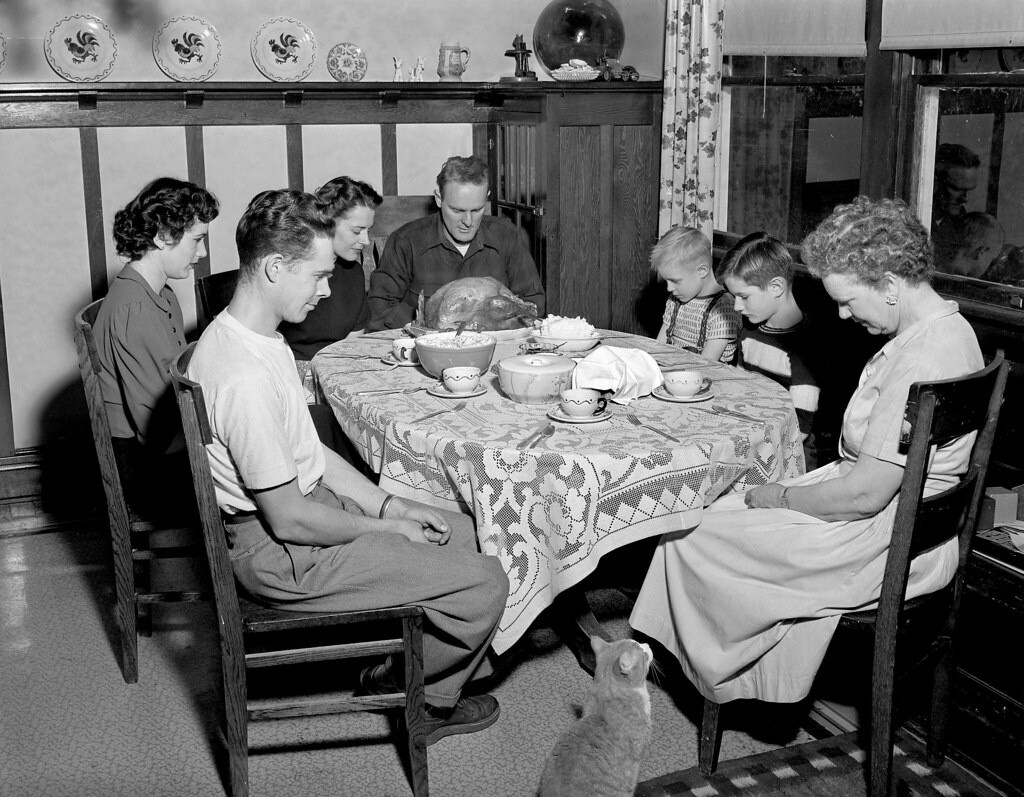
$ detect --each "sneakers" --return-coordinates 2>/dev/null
[426,693,500,747]
[360,663,405,694]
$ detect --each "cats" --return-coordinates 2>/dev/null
[536,634,654,797]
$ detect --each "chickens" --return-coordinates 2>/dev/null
[424,274,538,336]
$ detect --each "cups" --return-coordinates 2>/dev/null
[560,388,608,419]
[664,370,712,399]
[442,366,481,395]
[518,343,558,357]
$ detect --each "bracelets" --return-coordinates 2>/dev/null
[378,494,396,520]
[781,486,790,510]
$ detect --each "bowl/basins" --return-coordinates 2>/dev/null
[496,354,577,405]
[530,329,604,353]
[392,338,420,363]
[414,331,498,380]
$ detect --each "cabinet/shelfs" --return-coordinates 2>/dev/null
[921,519,1024,793]
[491,94,665,334]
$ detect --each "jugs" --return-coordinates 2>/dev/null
[437,41,471,83]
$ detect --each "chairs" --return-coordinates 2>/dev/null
[69,295,208,687]
[195,267,244,327]
[160,337,433,797]
[692,349,1012,797]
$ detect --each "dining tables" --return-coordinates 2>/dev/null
[303,318,805,692]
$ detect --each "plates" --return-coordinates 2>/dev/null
[152,14,224,82]
[327,43,368,82]
[547,404,614,423]
[427,381,488,398]
[381,351,421,367]
[0,29,9,74]
[44,12,119,82]
[251,16,318,83]
[651,384,715,403]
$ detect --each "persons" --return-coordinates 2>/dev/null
[931,143,1007,280]
[367,157,545,333]
[181,188,511,745]
[91,177,221,600]
[276,174,385,360]
[630,194,984,706]
